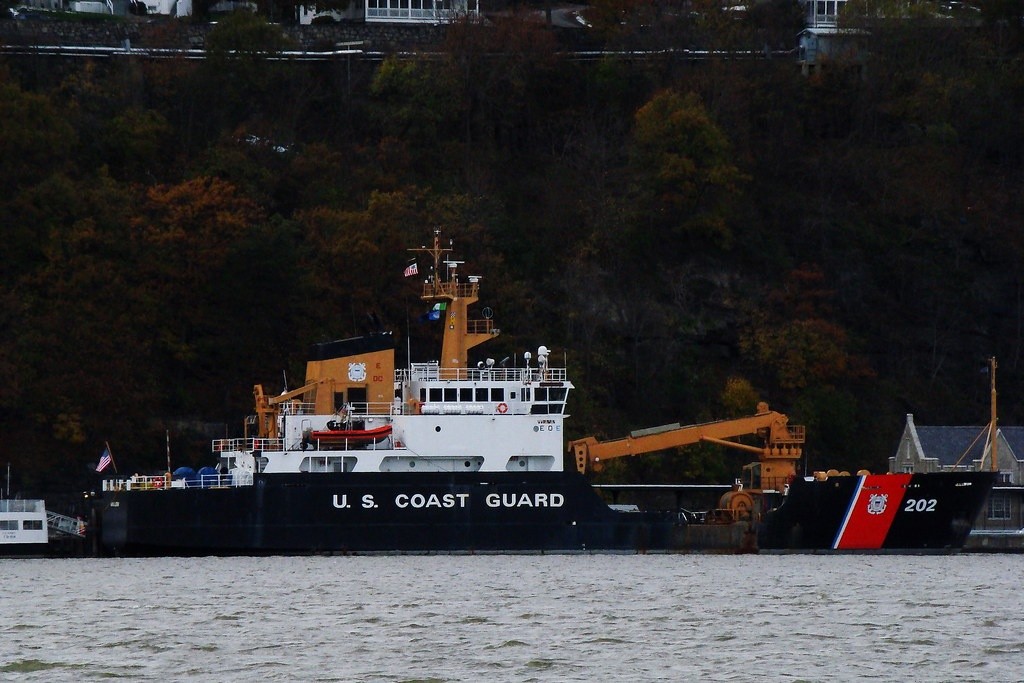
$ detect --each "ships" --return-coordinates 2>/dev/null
[92,229,1002,555]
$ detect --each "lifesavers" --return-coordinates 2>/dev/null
[153,477,162,488]
[498,403,508,413]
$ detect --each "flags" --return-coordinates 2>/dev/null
[428,311,441,320]
[95,449,111,473]
[403,263,418,278]
[432,302,446,310]
